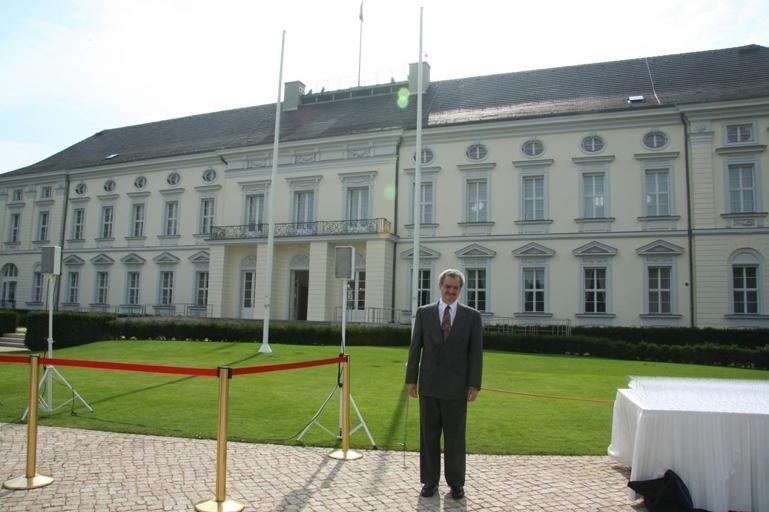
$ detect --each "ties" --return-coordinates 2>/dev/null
[441,306,451,342]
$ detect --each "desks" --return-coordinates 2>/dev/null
[607,377,769,512]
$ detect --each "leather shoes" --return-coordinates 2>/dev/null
[421,481,439,496]
[450,485,464,498]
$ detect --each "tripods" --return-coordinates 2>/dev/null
[297,285,379,451]
[20,284,94,422]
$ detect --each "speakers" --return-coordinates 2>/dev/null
[41,246,60,276]
[335,246,354,280]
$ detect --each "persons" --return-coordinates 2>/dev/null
[404,268,484,498]
